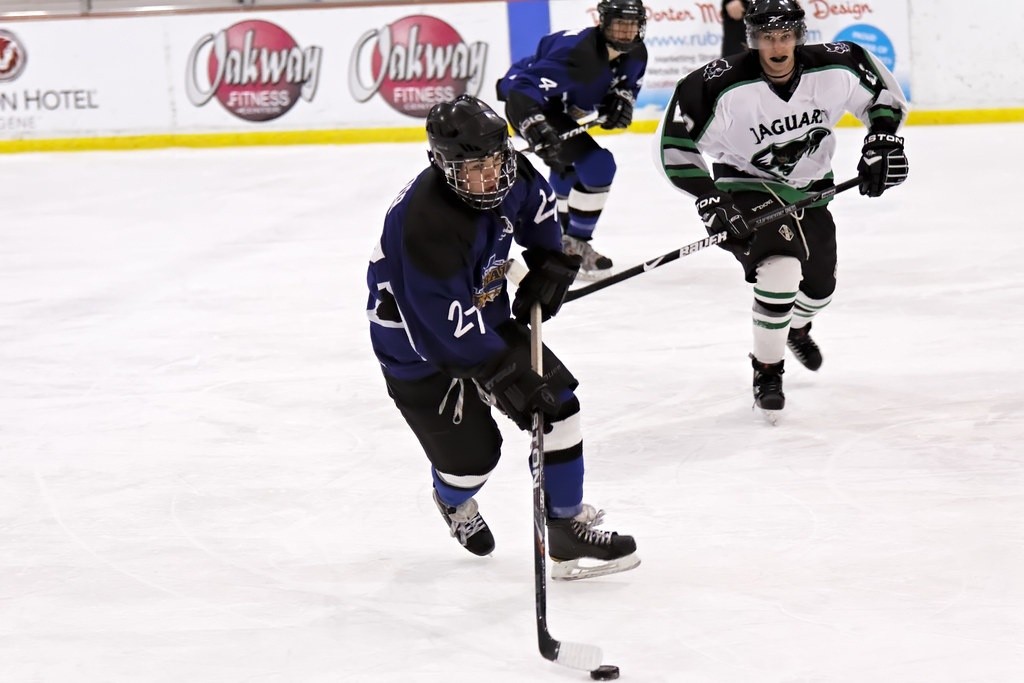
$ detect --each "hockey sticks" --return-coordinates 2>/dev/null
[521,114,608,158]
[527,304,620,671]
[562,175,861,297]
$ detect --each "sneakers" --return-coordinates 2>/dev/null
[542,509,640,580]
[580,242,612,281]
[431,490,495,556]
[788,322,823,374]
[749,356,791,418]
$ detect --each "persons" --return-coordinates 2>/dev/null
[653,1,909,426]
[366,94,641,582]
[721,1,751,58]
[497,1,648,282]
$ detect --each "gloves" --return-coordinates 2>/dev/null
[513,249,582,325]
[858,116,908,196]
[475,346,559,433]
[602,96,631,129]
[519,114,562,158]
[697,189,758,254]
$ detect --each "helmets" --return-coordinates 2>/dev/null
[744,0,805,25]
[427,94,516,209]
[597,0,649,52]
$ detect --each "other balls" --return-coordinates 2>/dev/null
[591,666,621,681]
